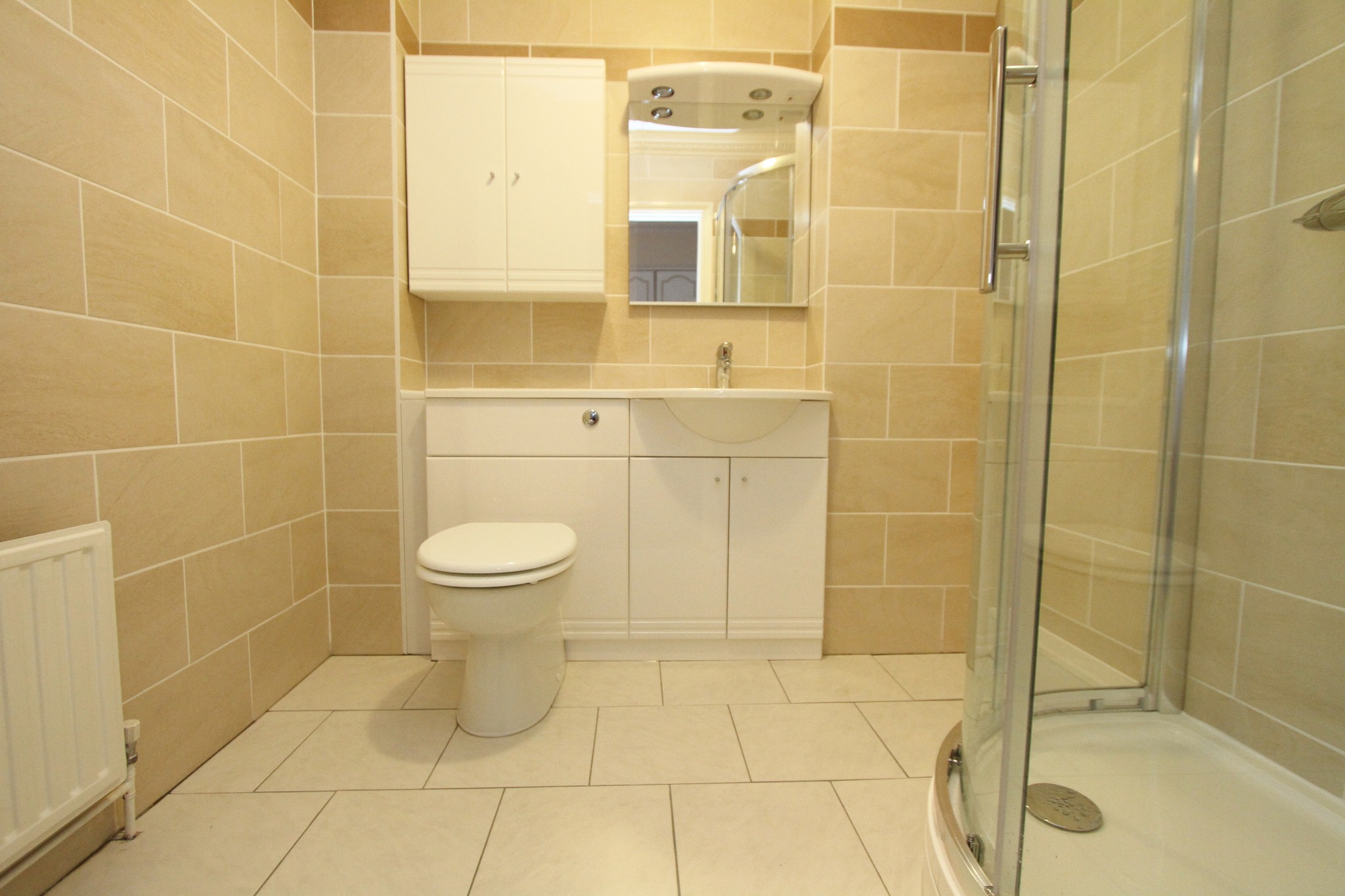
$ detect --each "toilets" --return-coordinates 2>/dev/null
[413,520,582,742]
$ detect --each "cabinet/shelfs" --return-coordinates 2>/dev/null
[425,397,829,664]
[405,55,608,305]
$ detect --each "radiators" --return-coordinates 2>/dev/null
[0,519,138,889]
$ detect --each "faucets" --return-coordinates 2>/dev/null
[716,340,733,388]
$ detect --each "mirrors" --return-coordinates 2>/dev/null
[628,98,805,305]
